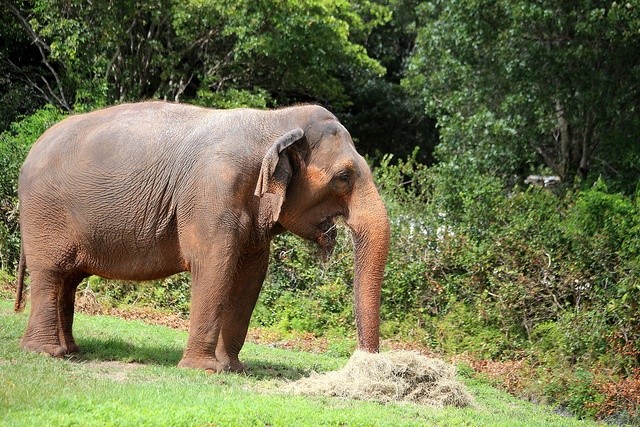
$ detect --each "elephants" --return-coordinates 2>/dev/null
[13,97,394,378]
